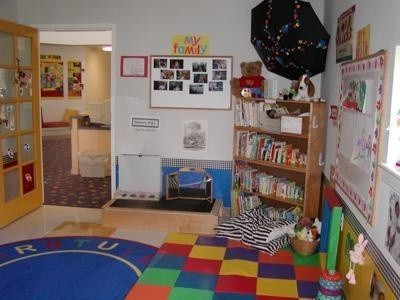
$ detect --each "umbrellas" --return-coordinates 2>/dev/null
[249,0,332,81]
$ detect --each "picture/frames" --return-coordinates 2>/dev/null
[120,56,147,77]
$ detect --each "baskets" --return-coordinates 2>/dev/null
[291,235,320,256]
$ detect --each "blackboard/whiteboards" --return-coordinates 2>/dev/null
[331,49,388,226]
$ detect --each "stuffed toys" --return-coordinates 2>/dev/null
[344,232,368,285]
[280,89,294,102]
[230,60,266,100]
[293,215,324,244]
[291,74,315,104]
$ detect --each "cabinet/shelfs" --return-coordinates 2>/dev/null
[233,97,327,220]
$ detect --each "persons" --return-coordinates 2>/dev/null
[154,58,227,94]
[370,271,385,300]
[336,15,352,45]
[385,200,400,253]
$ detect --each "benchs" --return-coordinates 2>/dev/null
[41,99,86,141]
[101,193,223,233]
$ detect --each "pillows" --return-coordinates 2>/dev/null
[63,108,78,123]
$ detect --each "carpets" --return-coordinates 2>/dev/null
[124,234,315,299]
[0,232,160,299]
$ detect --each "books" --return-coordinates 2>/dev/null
[262,78,278,100]
[231,99,310,222]
